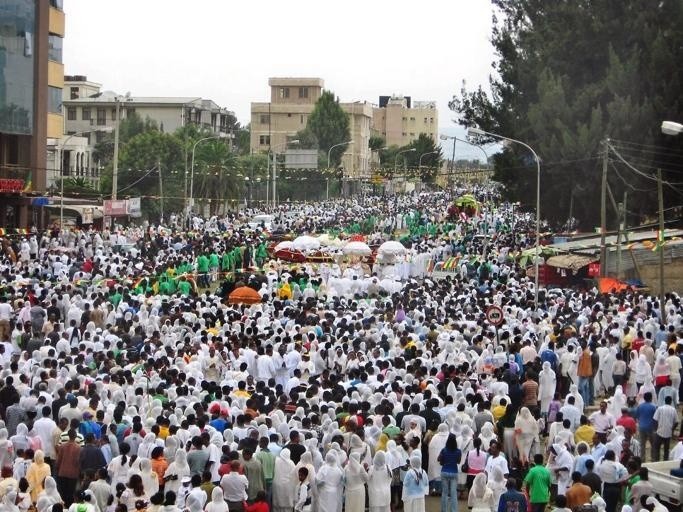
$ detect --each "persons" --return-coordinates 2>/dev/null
[1,185,683,512]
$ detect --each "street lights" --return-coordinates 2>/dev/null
[370,148,387,173]
[466,127,540,313]
[326,140,354,201]
[393,148,417,196]
[86,90,120,200]
[189,135,234,216]
[418,150,438,193]
[265,140,300,208]
[438,133,489,256]
[60,127,114,230]
[660,120,683,138]
[342,153,352,197]
